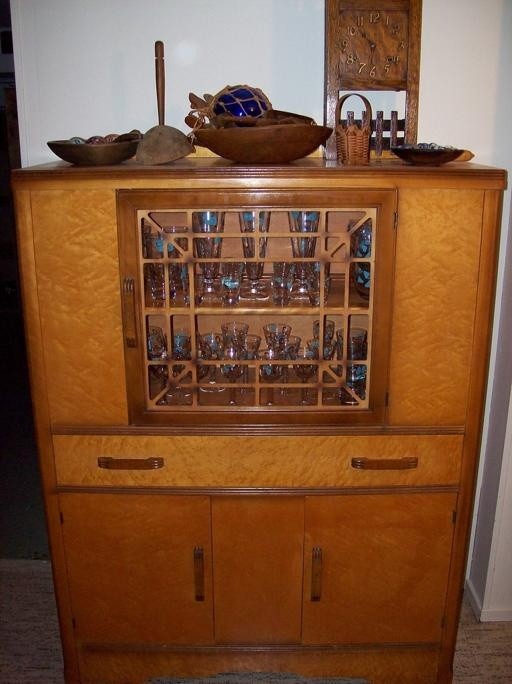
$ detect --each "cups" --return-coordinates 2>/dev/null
[239,211,271,300]
[193,212,225,302]
[221,262,244,306]
[337,327,368,404]
[271,210,331,307]
[143,226,198,305]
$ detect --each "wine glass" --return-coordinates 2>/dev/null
[147,319,337,406]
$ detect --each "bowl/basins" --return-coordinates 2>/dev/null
[391,144,465,166]
[192,123,333,166]
[47,140,140,167]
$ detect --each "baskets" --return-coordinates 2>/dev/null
[334,93,373,167]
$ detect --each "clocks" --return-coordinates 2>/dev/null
[324,0,422,90]
